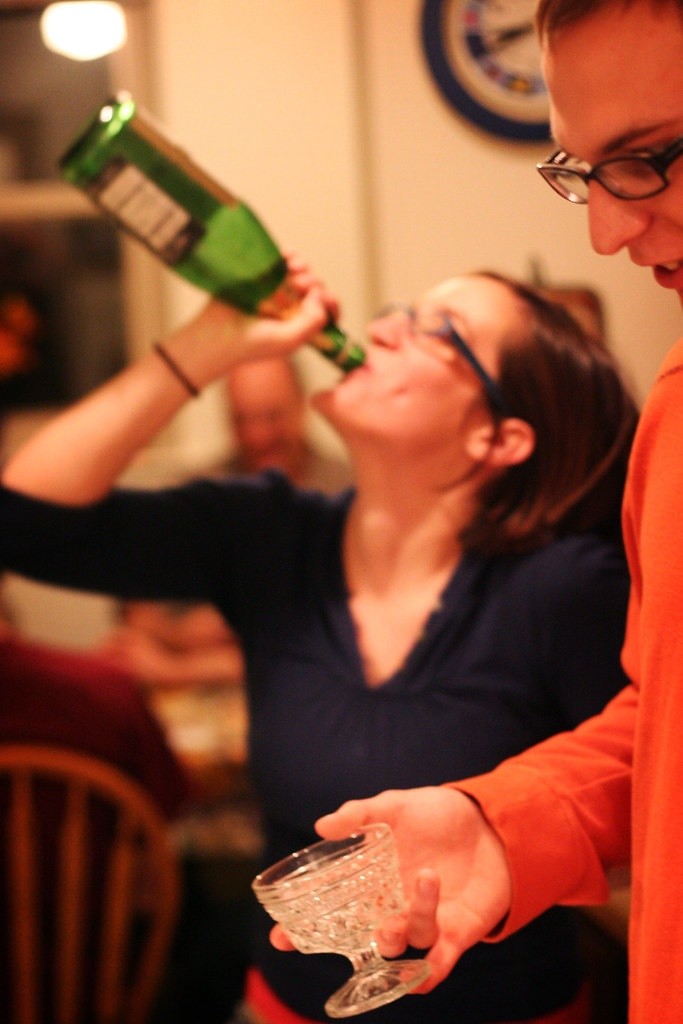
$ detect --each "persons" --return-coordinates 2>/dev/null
[0,257,638,1024]
[260,1,683,1024]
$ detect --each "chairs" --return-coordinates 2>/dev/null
[0,744,179,1024]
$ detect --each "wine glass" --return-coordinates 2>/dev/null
[251,822,434,1018]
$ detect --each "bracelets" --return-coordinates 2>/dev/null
[152,341,200,398]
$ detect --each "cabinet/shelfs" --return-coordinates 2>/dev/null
[0,0,159,361]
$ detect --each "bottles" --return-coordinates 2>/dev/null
[58,90,366,372]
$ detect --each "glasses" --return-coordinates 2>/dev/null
[537,133,683,206]
[390,304,506,420]
